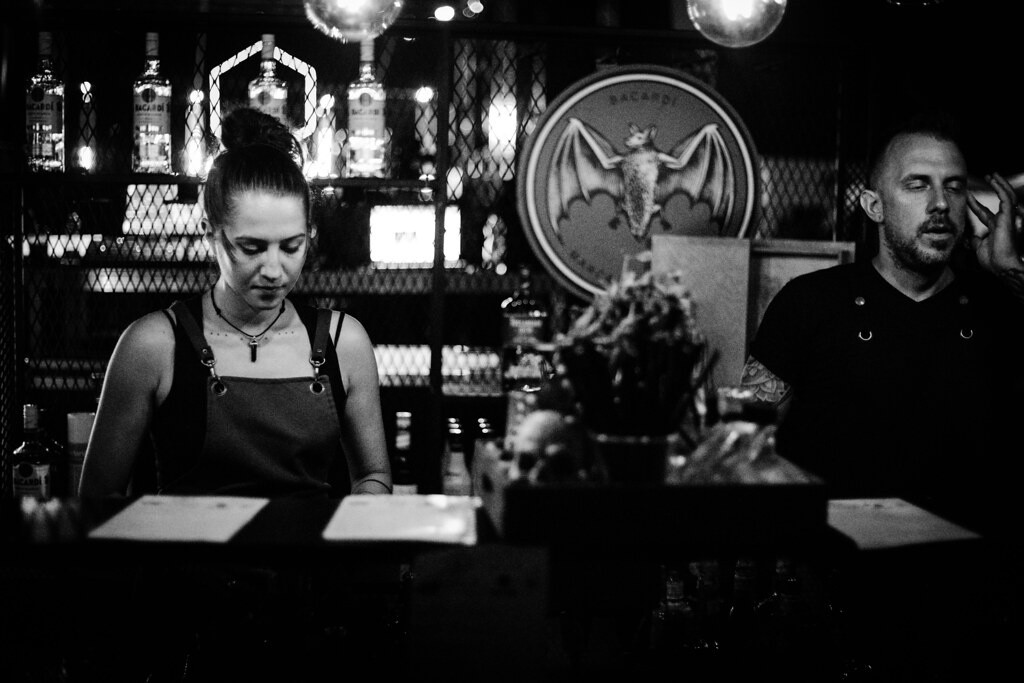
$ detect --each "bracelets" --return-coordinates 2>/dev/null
[352,479,392,493]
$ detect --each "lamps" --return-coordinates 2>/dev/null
[433,0,455,22]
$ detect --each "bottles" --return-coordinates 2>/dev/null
[132,31,173,174]
[390,411,416,496]
[347,36,391,178]
[10,403,52,496]
[248,33,290,132]
[25,30,67,173]
[444,417,511,495]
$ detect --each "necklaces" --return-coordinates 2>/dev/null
[210,284,285,362]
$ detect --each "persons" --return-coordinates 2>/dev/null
[78,106,392,500]
[735,126,1024,498]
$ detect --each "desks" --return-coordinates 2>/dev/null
[0,484,1024,683]
